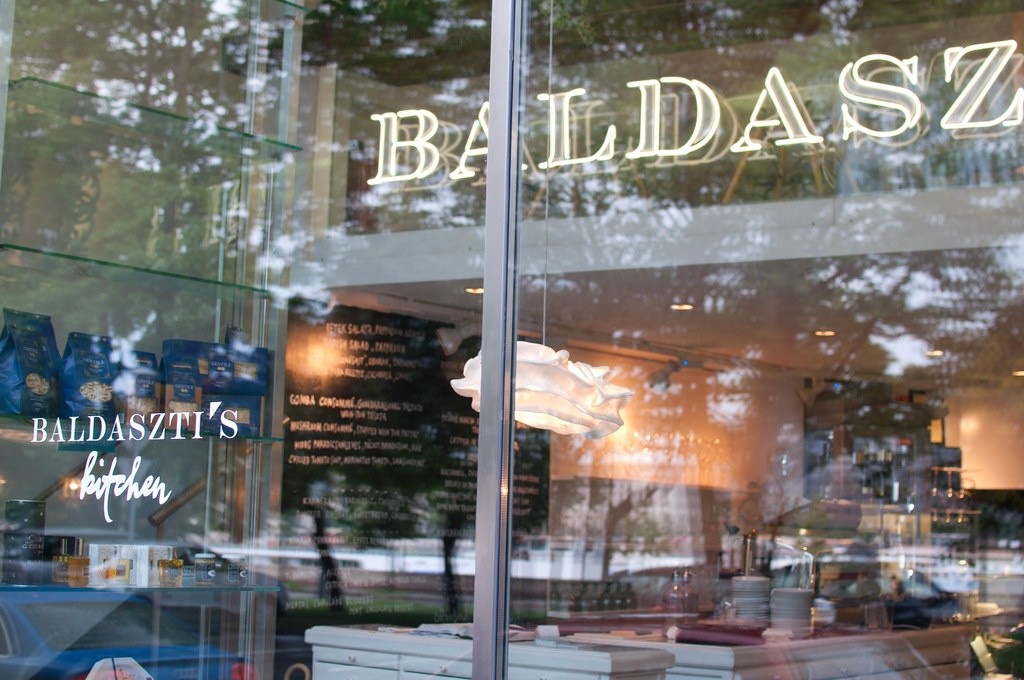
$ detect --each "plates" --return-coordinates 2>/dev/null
[769,589,815,640]
[730,575,770,625]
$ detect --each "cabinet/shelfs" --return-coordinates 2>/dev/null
[558,625,980,680]
[304,624,677,679]
[1,0,311,680]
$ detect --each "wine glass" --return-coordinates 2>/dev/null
[930,465,972,508]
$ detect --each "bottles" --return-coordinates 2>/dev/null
[665,566,699,629]
[855,444,912,505]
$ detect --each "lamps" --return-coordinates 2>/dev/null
[449,1,637,441]
